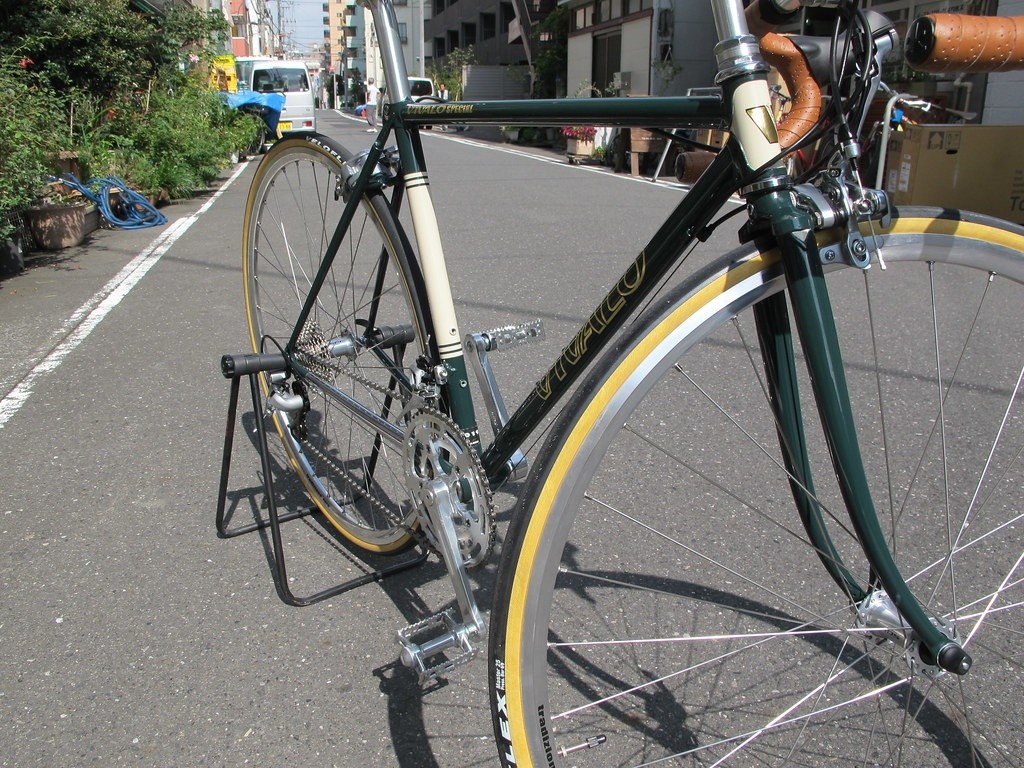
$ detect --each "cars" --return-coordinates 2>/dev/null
[354,104,370,119]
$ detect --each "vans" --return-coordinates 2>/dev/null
[381,76,437,130]
[248,61,317,139]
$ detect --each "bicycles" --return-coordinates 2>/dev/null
[210,1,1024,768]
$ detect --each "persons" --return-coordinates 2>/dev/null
[365,77,382,132]
[436,83,452,133]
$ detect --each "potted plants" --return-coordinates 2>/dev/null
[85,162,121,211]
[523,128,539,140]
[154,158,193,206]
[63,196,100,236]
[24,195,86,250]
[650,56,682,80]
[132,164,155,213]
[595,146,605,160]
[499,125,520,141]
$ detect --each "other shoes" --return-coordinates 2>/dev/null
[366,129,374,133]
[374,128,378,133]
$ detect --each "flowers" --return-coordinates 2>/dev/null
[559,126,597,146]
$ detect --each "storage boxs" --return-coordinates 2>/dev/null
[894,125,1023,224]
[711,130,730,147]
[885,131,903,203]
[694,129,711,153]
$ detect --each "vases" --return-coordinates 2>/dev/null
[566,138,595,155]
